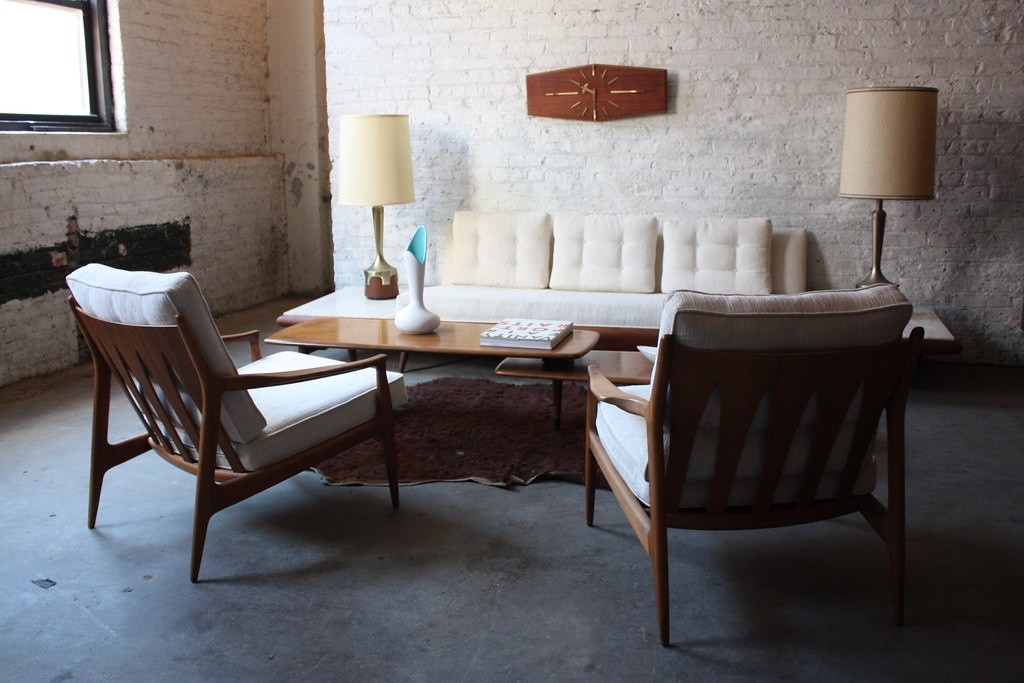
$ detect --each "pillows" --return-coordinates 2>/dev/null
[450,209,552,289]
[548,212,659,294]
[660,217,773,294]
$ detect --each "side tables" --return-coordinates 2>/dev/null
[275,285,408,363]
[903,305,963,356]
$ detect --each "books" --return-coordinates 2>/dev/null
[479,318,573,350]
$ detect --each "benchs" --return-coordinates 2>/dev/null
[395,222,808,374]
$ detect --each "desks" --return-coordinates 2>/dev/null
[264,316,600,433]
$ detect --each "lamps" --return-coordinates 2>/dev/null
[337,113,416,300]
[838,87,939,288]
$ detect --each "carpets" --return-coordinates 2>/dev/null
[310,376,612,490]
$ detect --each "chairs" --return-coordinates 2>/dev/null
[64,263,410,583]
[585,283,925,649]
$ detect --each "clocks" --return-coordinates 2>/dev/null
[526,64,668,122]
